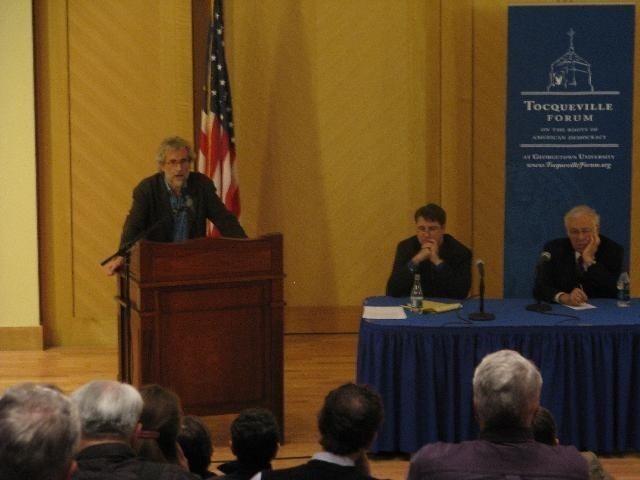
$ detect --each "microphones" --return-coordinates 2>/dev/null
[525,251,552,313]
[99,210,173,266]
[468,261,496,322]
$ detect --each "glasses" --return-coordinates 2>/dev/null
[163,158,191,166]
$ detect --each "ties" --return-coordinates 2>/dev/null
[577,255,585,273]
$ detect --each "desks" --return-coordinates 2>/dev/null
[355,292,639,459]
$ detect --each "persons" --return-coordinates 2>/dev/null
[386,203,473,301]
[406,348,590,480]
[532,204,624,307]
[1,379,385,480]
[107,135,246,278]
[531,404,615,480]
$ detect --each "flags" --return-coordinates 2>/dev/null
[196,2,241,239]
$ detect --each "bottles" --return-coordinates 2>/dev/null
[411,274,423,319]
[615,264,631,309]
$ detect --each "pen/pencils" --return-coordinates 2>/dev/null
[580,284,588,304]
